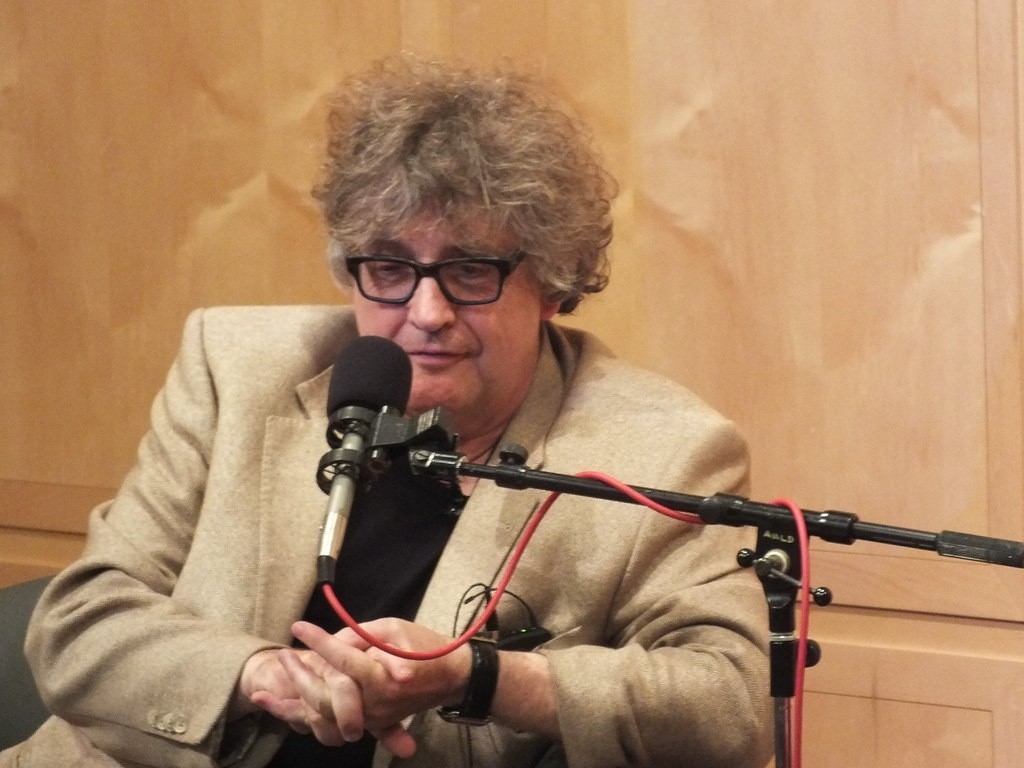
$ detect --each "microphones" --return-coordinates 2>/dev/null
[314,335,412,584]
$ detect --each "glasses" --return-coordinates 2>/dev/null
[343,246,527,307]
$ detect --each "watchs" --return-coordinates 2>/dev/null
[435,634,501,727]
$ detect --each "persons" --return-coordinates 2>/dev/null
[1,54,779,768]
